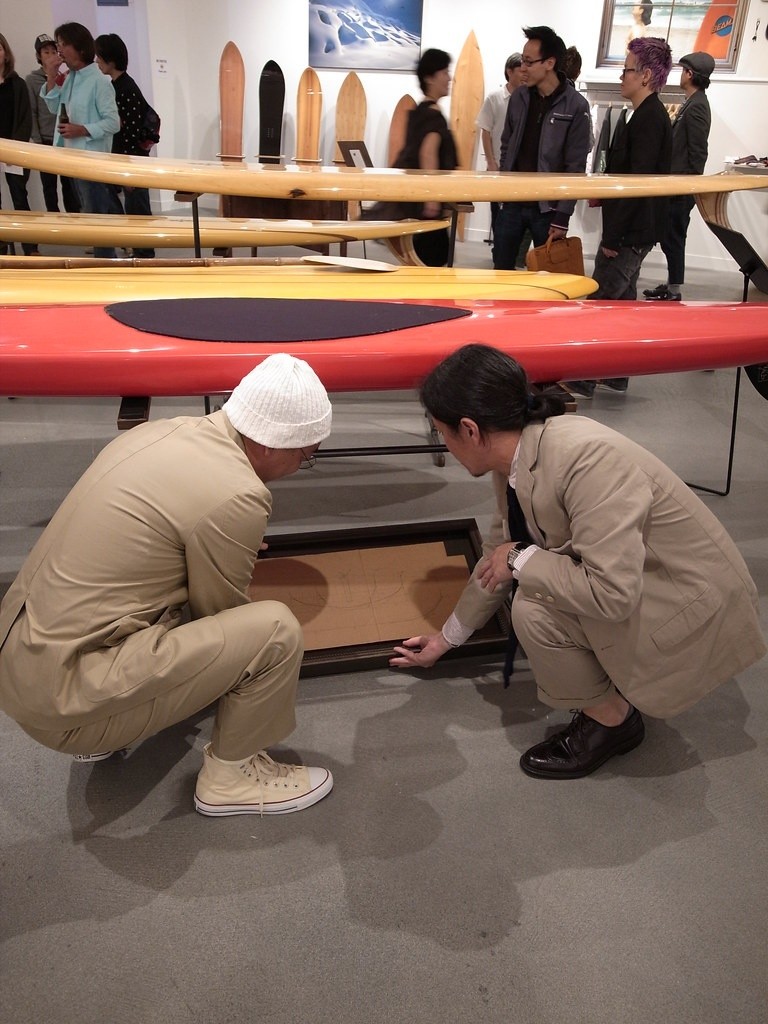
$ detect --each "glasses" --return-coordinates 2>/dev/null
[520,57,546,67]
[622,69,639,77]
[298,447,316,469]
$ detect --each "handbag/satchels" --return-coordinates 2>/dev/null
[526,233,584,277]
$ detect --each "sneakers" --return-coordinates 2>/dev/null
[193,743,334,819]
[72,750,114,762]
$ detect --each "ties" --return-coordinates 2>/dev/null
[502,483,527,688]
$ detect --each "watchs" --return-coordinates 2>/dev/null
[506,542,531,571]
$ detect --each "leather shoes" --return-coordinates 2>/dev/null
[519,700,645,781]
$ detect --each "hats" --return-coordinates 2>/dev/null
[222,353,332,449]
[34,34,55,53]
[679,51,717,78]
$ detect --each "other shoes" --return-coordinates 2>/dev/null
[594,377,629,393]
[556,380,596,400]
[642,284,682,301]
[29,252,43,256]
[85,246,94,255]
[126,247,135,258]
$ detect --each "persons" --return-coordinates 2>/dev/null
[0,32,39,256]
[475,53,523,230]
[389,344,768,781]
[94,34,160,259]
[24,33,81,213]
[40,22,121,258]
[559,37,673,400]
[626,0,653,42]
[643,52,715,300]
[491,25,591,272]
[398,49,463,267]
[515,46,596,268]
[0,353,334,816]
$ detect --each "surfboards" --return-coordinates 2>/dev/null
[219,27,486,243]
[0,137,767,398]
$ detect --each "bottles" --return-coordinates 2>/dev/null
[59,103,69,133]
